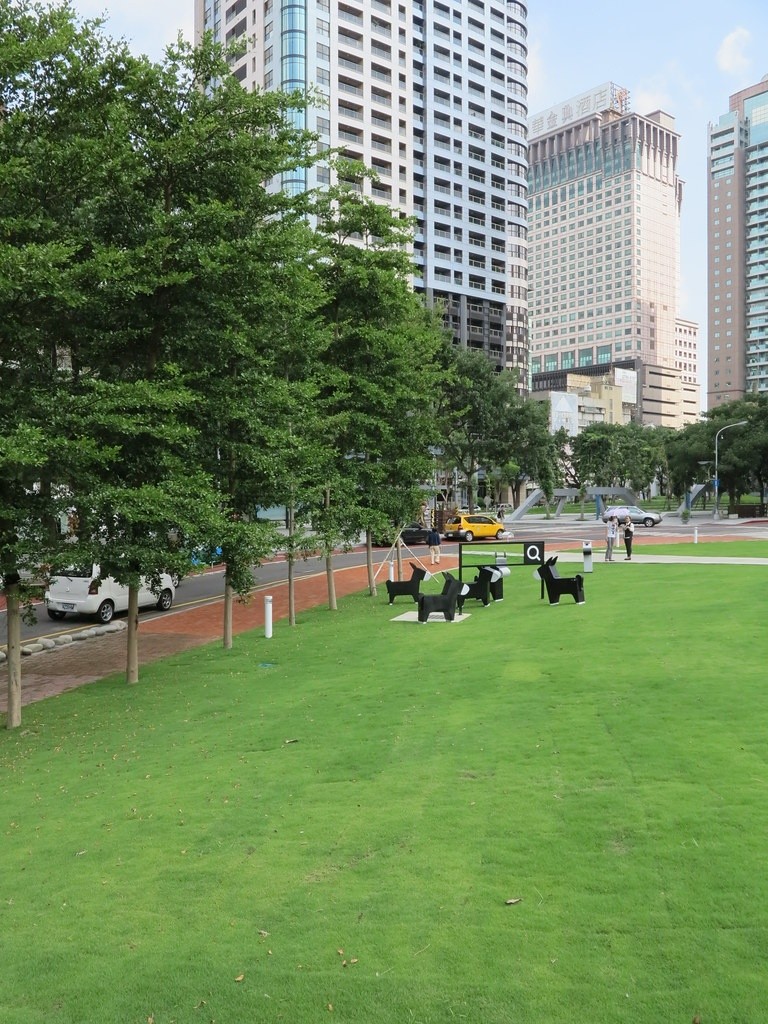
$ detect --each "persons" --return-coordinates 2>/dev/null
[604,516,618,561]
[426,528,441,565]
[620,515,634,560]
[499,505,506,524]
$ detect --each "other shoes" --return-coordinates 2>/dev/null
[605,559,609,561]
[610,559,614,561]
[625,557,631,560]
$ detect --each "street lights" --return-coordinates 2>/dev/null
[713,420,749,519]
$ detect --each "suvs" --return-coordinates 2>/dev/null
[443,514,506,542]
[602,506,663,527]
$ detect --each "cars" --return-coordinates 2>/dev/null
[376,519,432,547]
[45,552,175,623]
